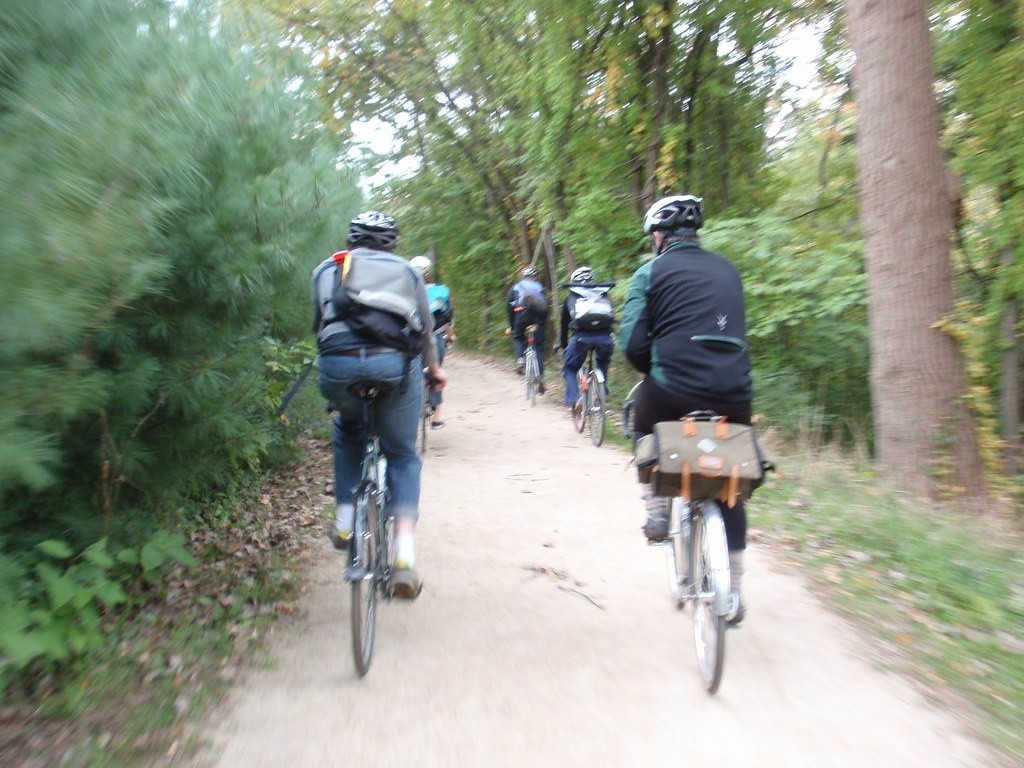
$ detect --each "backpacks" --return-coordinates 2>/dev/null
[560,282,616,330]
[518,280,548,316]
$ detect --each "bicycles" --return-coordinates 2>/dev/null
[550,342,610,448]
[421,334,454,454]
[322,369,442,680]
[620,378,744,698]
[506,322,547,406]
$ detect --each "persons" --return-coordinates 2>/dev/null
[615,194,752,624]
[506,265,550,394]
[310,209,457,598]
[560,267,614,416]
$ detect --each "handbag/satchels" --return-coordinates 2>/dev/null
[635,410,766,510]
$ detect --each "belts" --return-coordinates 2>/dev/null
[338,346,400,354]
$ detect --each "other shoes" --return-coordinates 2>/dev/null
[728,599,744,623]
[517,358,525,375]
[432,421,444,429]
[390,556,424,600]
[644,516,669,541]
[572,397,582,421]
[331,512,354,550]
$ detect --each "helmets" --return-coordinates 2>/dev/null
[521,267,542,277]
[569,266,595,284]
[347,210,400,250]
[409,255,433,277]
[642,195,704,236]
